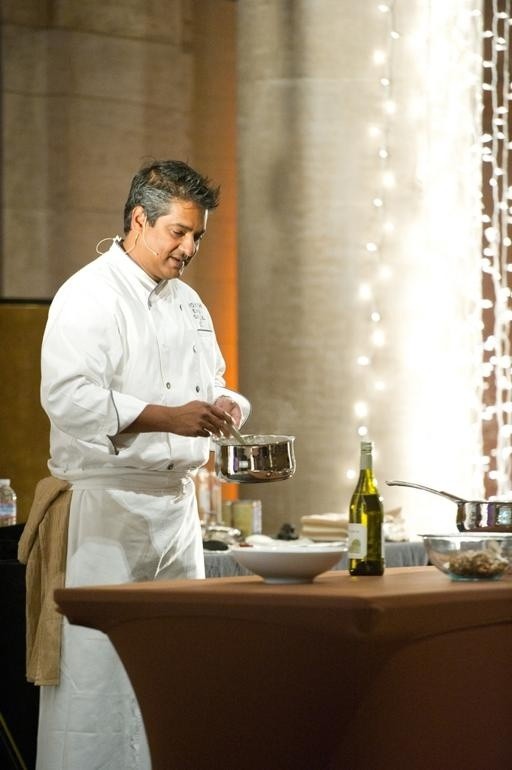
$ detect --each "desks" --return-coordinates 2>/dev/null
[53,575,512,770]
[199,540,430,577]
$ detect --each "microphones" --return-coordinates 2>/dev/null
[143,216,159,257]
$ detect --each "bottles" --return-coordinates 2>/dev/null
[346,440,385,577]
[0,478,18,528]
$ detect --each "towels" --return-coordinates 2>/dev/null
[16,476,72,688]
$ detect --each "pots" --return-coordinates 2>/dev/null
[213,434,297,485]
[386,480,511,533]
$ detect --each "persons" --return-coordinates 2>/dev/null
[17,158,252,770]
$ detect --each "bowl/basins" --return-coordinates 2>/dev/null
[419,534,512,583]
[229,548,349,586]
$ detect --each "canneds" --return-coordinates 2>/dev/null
[221,500,263,541]
